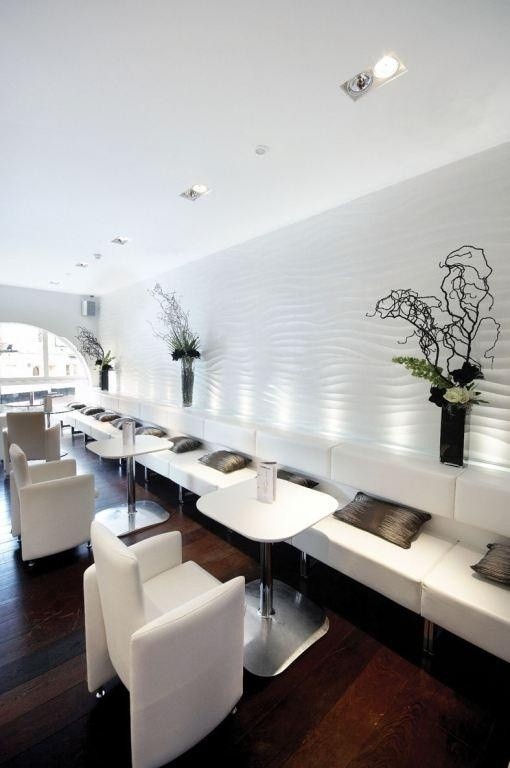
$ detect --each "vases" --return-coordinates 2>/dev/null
[99,370,108,390]
[438,405,472,471]
[180,356,194,406]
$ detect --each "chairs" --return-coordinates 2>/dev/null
[2,410,61,479]
[82,520,245,768]
[9,442,95,567]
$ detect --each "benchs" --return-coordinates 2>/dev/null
[64,385,509,658]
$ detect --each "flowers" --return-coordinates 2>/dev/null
[76,326,114,373]
[151,282,201,362]
[366,243,501,405]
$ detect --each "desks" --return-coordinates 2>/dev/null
[3,400,47,411]
[29,406,74,458]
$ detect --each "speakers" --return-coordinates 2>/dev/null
[81,301,96,317]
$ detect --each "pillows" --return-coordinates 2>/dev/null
[135,426,166,438]
[168,434,203,454]
[333,488,430,549]
[471,539,509,586]
[67,401,86,409]
[275,469,319,487]
[109,417,144,430]
[198,449,252,473]
[78,406,104,415]
[93,411,122,422]
